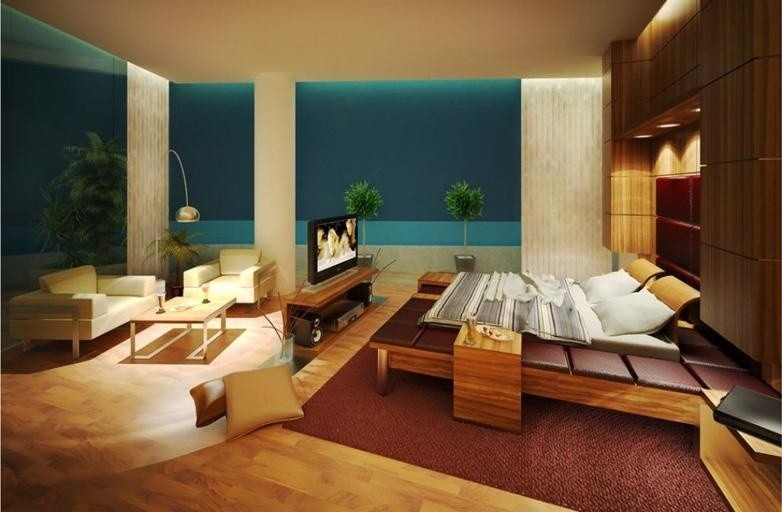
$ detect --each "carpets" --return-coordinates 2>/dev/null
[282,285,735,511]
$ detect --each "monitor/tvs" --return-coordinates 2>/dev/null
[308,214,358,285]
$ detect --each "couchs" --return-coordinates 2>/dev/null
[184,248,277,312]
[8,265,165,359]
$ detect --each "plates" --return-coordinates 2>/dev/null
[475,325,510,341]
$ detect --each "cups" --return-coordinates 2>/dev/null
[157,292,165,313]
[202,285,209,303]
[465,317,479,344]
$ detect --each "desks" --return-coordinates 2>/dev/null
[454,322,521,432]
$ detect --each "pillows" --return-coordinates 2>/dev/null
[584,267,642,304]
[590,289,674,335]
[189,376,226,427]
[224,364,304,441]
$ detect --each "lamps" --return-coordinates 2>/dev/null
[168,151,200,222]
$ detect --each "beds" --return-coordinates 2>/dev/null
[369,258,782,427]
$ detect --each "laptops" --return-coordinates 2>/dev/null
[713,386,782,448]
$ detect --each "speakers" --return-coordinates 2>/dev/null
[290,310,323,348]
[347,283,373,308]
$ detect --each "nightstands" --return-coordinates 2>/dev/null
[698,389,782,511]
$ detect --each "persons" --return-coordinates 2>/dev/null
[340,219,355,255]
[318,228,340,262]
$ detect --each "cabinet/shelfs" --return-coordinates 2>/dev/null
[699,56,781,395]
[417,271,458,293]
[616,0,780,139]
[602,39,653,260]
[283,266,378,352]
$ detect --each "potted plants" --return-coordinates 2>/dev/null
[445,180,485,272]
[345,181,382,266]
